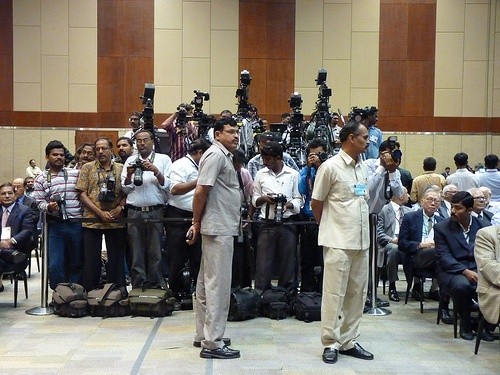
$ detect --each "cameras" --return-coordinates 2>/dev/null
[129,159,146,186]
[241,203,249,217]
[387,136,402,160]
[98,176,116,202]
[314,151,328,162]
[55,198,68,222]
[271,193,287,224]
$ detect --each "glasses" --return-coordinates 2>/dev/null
[219,129,239,135]
[263,144,278,157]
[473,197,485,200]
[95,146,110,151]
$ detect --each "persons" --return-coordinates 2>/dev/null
[233,132,306,296]
[297,138,328,292]
[311,121,374,364]
[441,167,450,178]
[329,112,343,156]
[124,112,143,139]
[162,104,232,162]
[26,159,42,178]
[364,140,500,341]
[0,177,41,292]
[186,116,241,360]
[121,130,210,302]
[34,136,128,299]
[241,105,290,160]
[348,107,382,159]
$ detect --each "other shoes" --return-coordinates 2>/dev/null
[11,250,28,263]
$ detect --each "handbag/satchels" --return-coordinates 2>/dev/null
[52,250,175,317]
[226,286,261,322]
[292,291,322,323]
[255,286,295,320]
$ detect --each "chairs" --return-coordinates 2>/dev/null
[1,213,44,308]
[375,222,500,354]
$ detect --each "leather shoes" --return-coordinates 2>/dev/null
[323,348,338,364]
[389,289,399,301]
[339,342,373,360]
[365,296,390,307]
[200,345,240,359]
[478,327,494,342]
[428,289,440,301]
[440,308,453,324]
[411,288,424,301]
[460,321,474,340]
[193,337,231,347]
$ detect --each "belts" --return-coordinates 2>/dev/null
[128,203,166,212]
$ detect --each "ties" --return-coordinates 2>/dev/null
[399,207,404,227]
[428,218,432,235]
[2,209,9,228]
[15,199,19,203]
